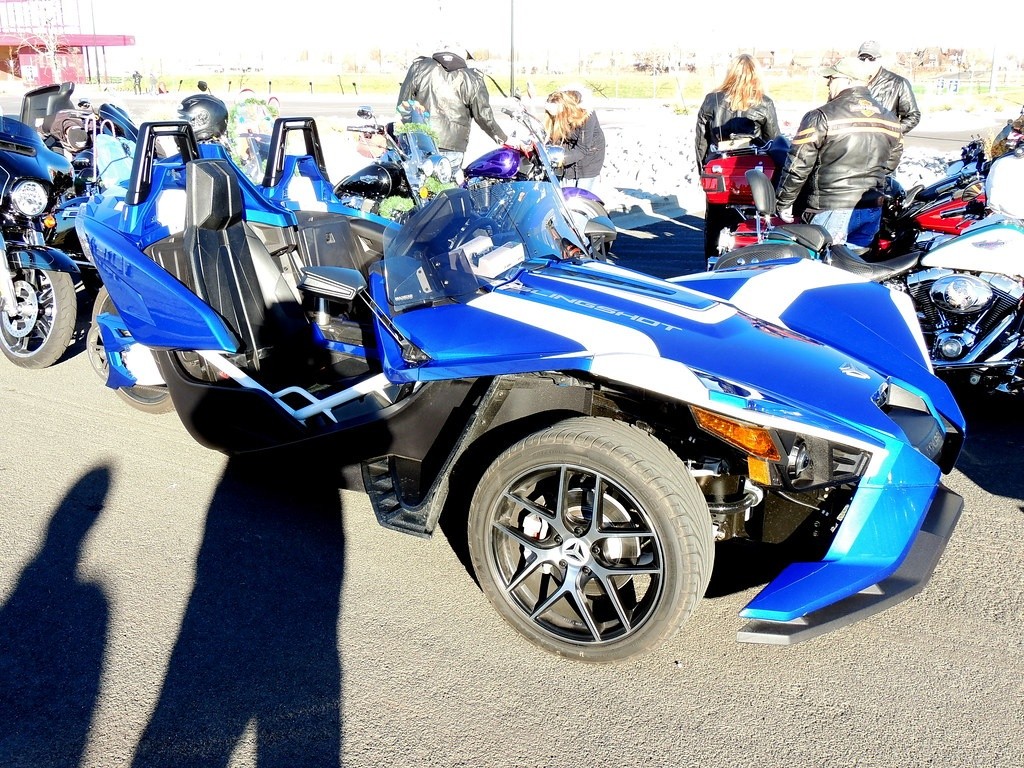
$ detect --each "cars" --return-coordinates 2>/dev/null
[71,111,966,664]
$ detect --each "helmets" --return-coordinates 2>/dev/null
[985,155,1024,220]
[173,94,228,148]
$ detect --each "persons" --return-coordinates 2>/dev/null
[150,74,156,91]
[545,90,604,191]
[695,54,782,267]
[858,41,920,135]
[777,57,902,248]
[133,71,142,95]
[396,42,526,177]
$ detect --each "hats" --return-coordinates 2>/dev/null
[857,39,885,61]
[818,56,870,84]
[444,39,476,62]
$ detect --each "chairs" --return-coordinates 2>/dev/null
[182,157,378,409]
[294,214,373,316]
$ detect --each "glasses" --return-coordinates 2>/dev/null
[858,54,881,61]
[827,75,850,85]
[547,97,561,104]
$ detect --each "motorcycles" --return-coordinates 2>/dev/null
[693,133,993,263]
[879,133,987,203]
[0,115,84,370]
[718,152,1023,406]
[74,102,272,195]
